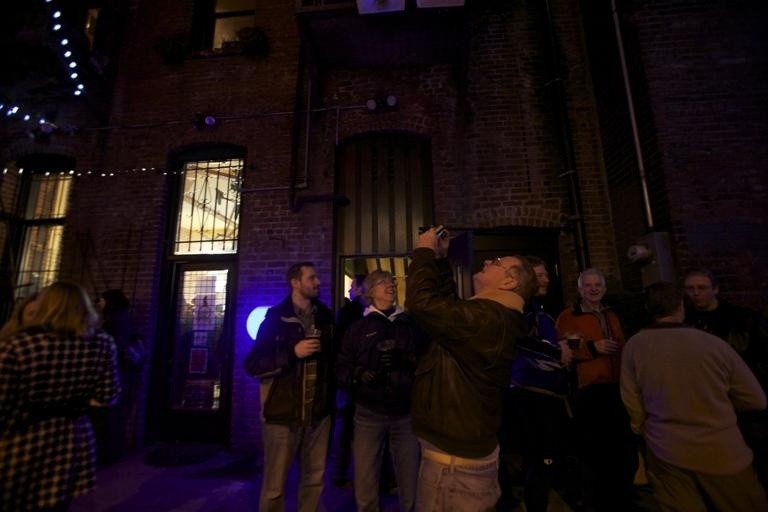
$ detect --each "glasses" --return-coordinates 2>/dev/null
[493,258,513,279]
[368,278,398,292]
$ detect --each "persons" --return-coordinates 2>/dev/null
[234,221,767,512]
[0,292,40,341]
[1,279,124,512]
[95,287,132,465]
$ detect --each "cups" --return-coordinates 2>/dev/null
[303,327,320,338]
[567,332,580,350]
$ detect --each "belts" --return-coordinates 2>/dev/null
[423,448,501,467]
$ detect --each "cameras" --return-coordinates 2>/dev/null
[437,229,448,239]
